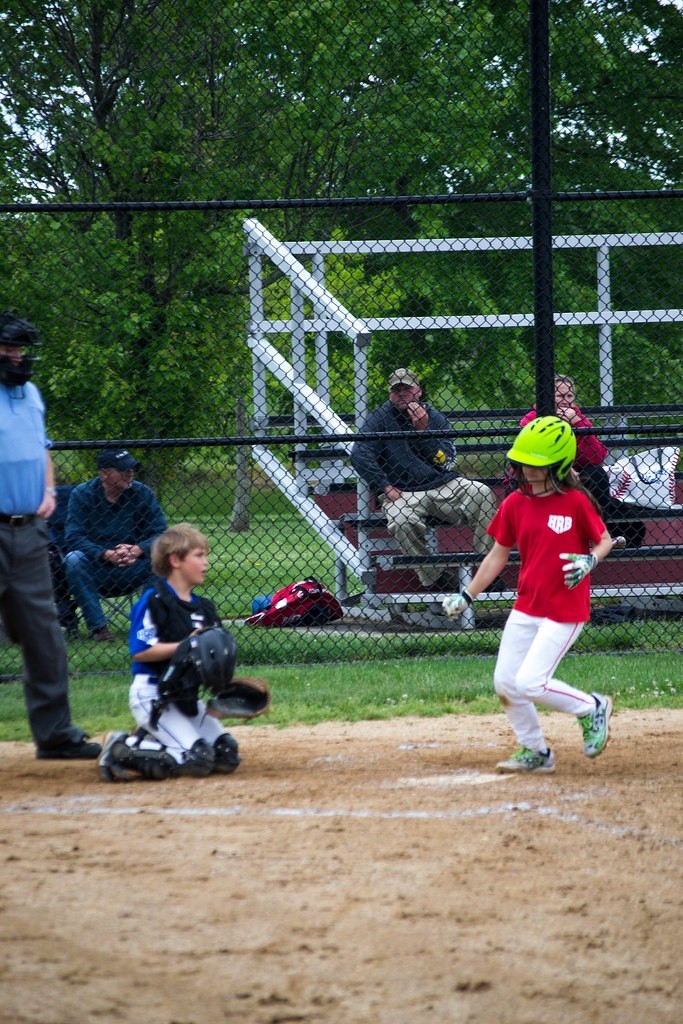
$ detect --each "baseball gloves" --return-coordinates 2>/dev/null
[206,678,271,718]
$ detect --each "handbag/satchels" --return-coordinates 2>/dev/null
[247,576,343,626]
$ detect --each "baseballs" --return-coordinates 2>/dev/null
[451,602,461,614]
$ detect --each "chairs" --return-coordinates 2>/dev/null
[50,484,151,639]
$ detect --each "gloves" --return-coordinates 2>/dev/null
[442,592,472,622]
[560,551,598,590]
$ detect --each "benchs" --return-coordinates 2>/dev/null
[266,402,683,600]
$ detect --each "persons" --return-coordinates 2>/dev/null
[503,375,612,522]
[96,522,240,784]
[0,313,102,759]
[61,447,167,643]
[440,416,614,776]
[351,367,505,615]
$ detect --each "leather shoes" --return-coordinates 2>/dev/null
[92,626,115,642]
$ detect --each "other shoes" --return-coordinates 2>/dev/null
[485,576,507,592]
[426,575,460,591]
[613,535,625,547]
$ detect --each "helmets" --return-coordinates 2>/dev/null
[506,416,577,480]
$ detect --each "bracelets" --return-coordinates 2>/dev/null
[45,487,57,496]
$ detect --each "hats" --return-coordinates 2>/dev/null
[388,369,419,389]
[97,449,142,473]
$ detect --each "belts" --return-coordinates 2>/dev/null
[1,510,39,529]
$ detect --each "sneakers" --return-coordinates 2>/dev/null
[577,692,614,758]
[36,737,101,760]
[495,744,556,774]
[98,728,129,783]
